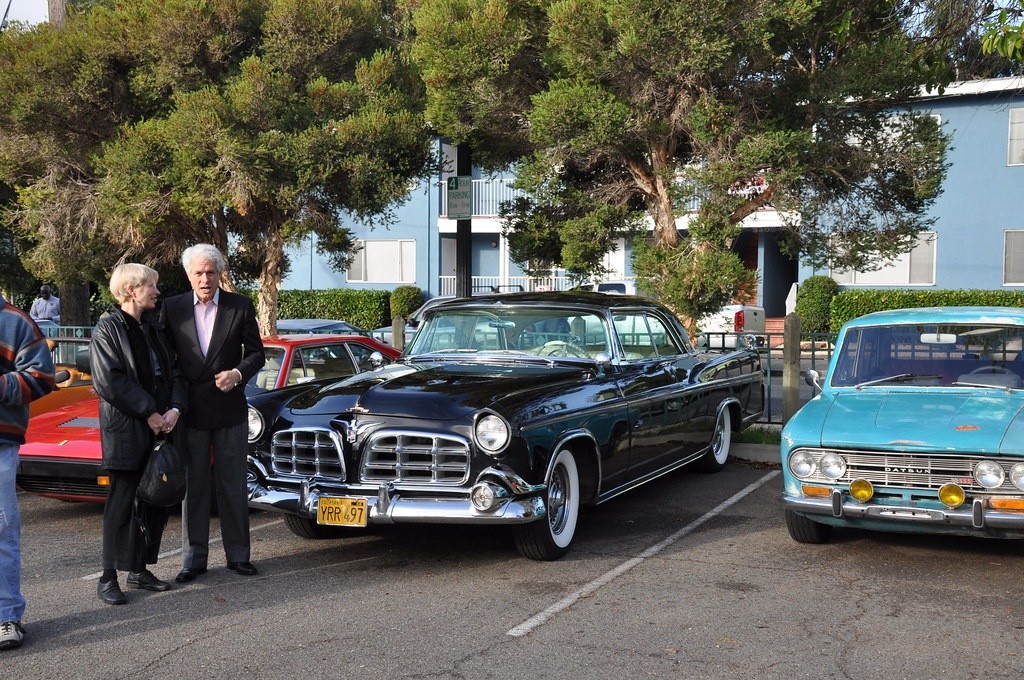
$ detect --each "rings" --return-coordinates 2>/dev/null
[224,385,226,390]
[167,427,170,430]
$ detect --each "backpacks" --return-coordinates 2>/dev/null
[134,434,186,546]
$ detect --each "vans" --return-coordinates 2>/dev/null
[565,282,626,321]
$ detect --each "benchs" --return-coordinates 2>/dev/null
[523,344,672,360]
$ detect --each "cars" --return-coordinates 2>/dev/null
[780,306,1024,544]
[19,330,403,503]
[276,319,381,343]
[45,337,93,387]
[247,291,765,561]
[373,295,571,350]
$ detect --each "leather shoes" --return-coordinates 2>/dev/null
[227,562,257,575]
[176,567,207,583]
[127,569,171,590]
[97,577,127,604]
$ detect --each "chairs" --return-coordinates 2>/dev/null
[256,357,303,390]
[324,357,353,373]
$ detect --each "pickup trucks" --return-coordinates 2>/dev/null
[567,295,765,348]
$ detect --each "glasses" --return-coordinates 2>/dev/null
[144,282,156,289]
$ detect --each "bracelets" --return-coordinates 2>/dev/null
[172,408,181,416]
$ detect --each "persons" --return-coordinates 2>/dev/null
[30,285,60,326]
[0,294,56,652]
[89,263,187,605]
[155,244,265,584]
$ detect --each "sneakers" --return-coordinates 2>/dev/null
[0,621,25,649]
